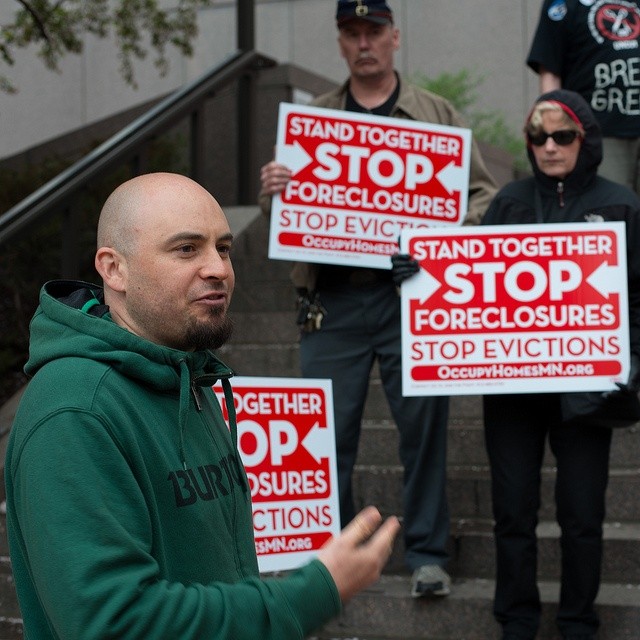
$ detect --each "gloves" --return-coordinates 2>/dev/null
[390,253,420,288]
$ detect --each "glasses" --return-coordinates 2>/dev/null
[526,128,584,147]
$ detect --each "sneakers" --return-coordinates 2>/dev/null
[409,564,452,599]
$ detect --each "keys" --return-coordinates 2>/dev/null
[295,290,328,338]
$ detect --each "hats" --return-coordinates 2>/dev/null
[335,1,394,30]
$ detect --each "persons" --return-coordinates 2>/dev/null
[257,0,500,599]
[3,172,402,640]
[390,88,640,640]
[525,0,640,193]
